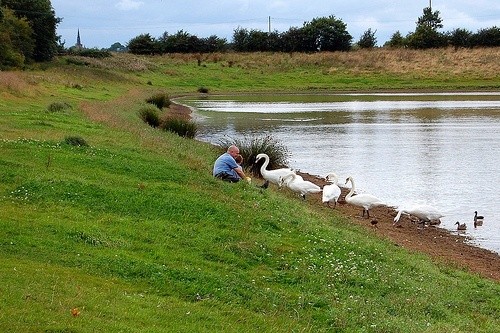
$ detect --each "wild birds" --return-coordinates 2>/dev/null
[474,211,484,221]
[455,222,467,230]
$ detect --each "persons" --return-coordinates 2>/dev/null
[234,154,243,171]
[213,145,248,183]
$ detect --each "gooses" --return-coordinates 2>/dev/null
[278,172,323,201]
[392,203,443,229]
[345,176,387,220]
[322,174,341,210]
[255,153,297,189]
